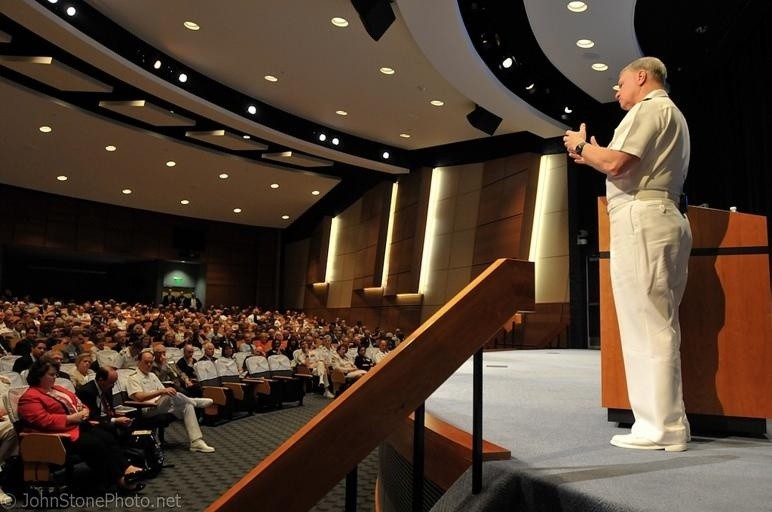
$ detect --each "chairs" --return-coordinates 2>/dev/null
[2,347,345,488]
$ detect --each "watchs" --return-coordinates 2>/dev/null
[575,142,588,156]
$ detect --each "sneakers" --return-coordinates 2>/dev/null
[0,489,13,504]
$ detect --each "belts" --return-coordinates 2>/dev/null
[607,190,681,211]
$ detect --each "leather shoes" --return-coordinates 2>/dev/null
[323,392,335,398]
[190,440,215,453]
[610,434,687,452]
[118,468,151,492]
[194,398,214,408]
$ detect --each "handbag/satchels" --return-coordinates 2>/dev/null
[124,432,164,478]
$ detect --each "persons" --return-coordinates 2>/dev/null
[0,287,406,506]
[563,55,693,452]
